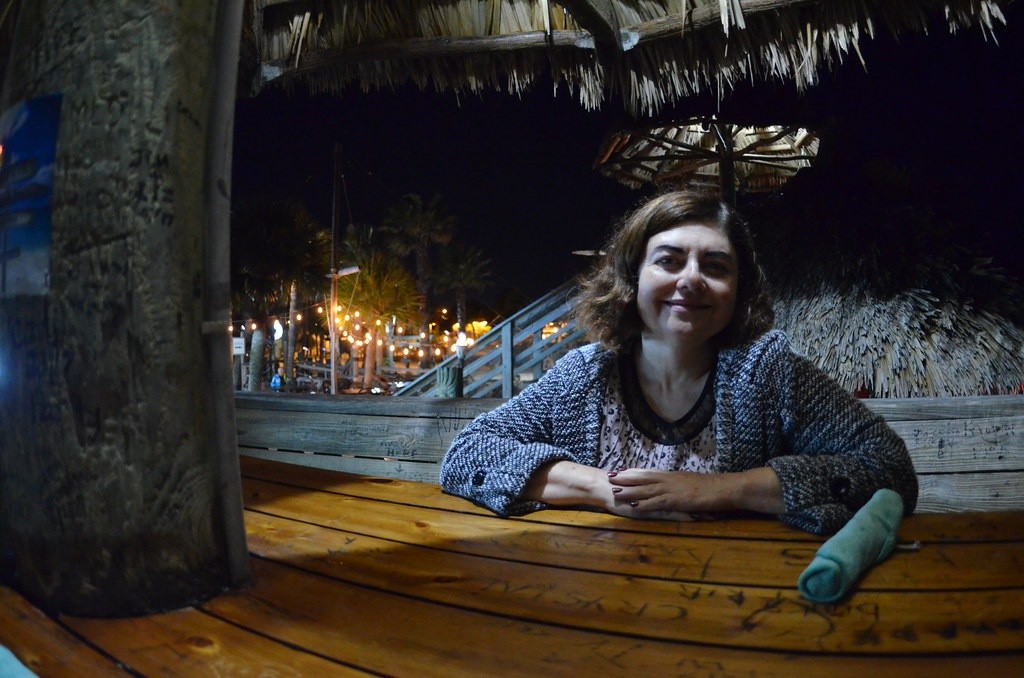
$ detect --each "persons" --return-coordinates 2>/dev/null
[435,188,921,535]
[271,367,292,394]
